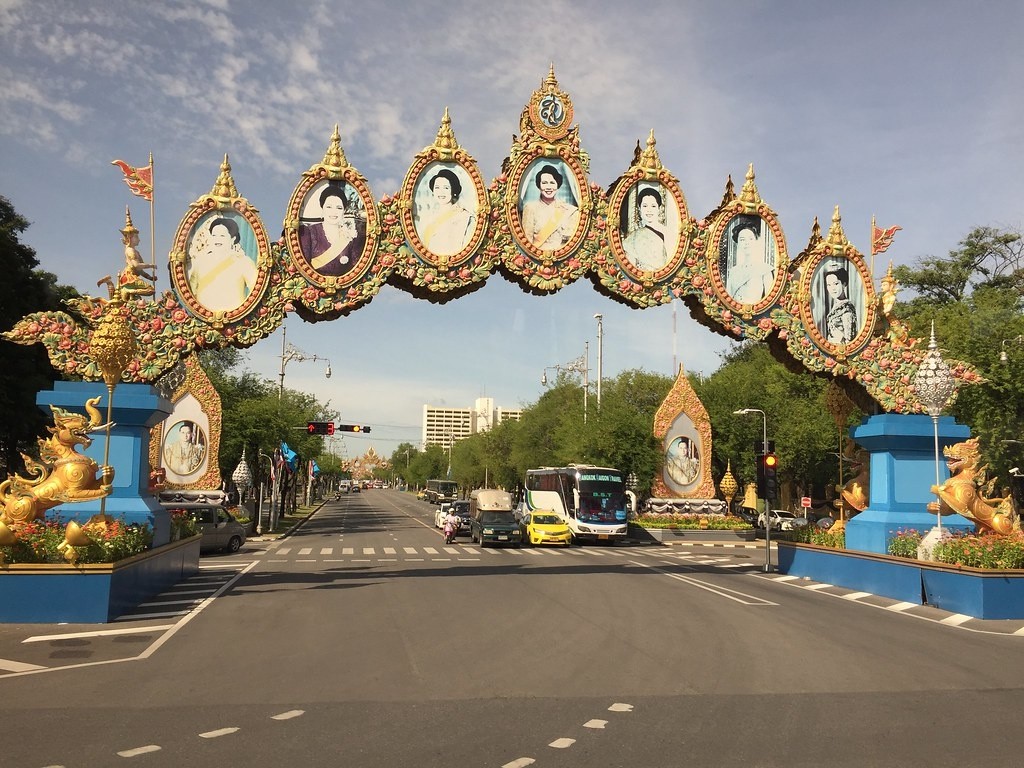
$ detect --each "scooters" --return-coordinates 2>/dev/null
[442,518,460,544]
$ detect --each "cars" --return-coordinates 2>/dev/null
[519,511,573,547]
[435,502,460,530]
[417,489,427,501]
[733,506,760,529]
[333,479,389,502]
[757,510,802,533]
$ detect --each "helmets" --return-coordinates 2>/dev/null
[449,508,454,513]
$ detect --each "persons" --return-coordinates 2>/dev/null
[823,261,857,343]
[667,441,699,484]
[416,169,476,258]
[165,425,202,473]
[189,218,259,311]
[97,205,159,303]
[443,508,458,539]
[725,223,775,305]
[874,259,925,350]
[522,165,580,251]
[621,188,677,273]
[298,186,362,276]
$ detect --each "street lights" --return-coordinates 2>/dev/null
[733,408,769,573]
[541,340,594,425]
[277,324,333,414]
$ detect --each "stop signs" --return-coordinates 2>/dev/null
[801,498,811,507]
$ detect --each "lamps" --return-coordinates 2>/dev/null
[55,519,92,566]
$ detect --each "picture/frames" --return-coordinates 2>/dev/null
[504,143,593,266]
[283,169,380,296]
[664,435,701,488]
[705,206,789,320]
[162,420,207,477]
[606,170,692,288]
[796,246,878,363]
[171,199,270,330]
[400,152,491,273]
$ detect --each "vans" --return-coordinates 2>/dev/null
[469,488,523,549]
[158,504,248,554]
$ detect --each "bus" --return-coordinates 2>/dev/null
[522,462,637,549]
[426,480,462,504]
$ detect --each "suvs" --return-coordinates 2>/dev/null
[446,499,472,537]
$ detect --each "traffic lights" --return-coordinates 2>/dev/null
[340,424,360,432]
[307,420,334,436]
[764,454,777,500]
